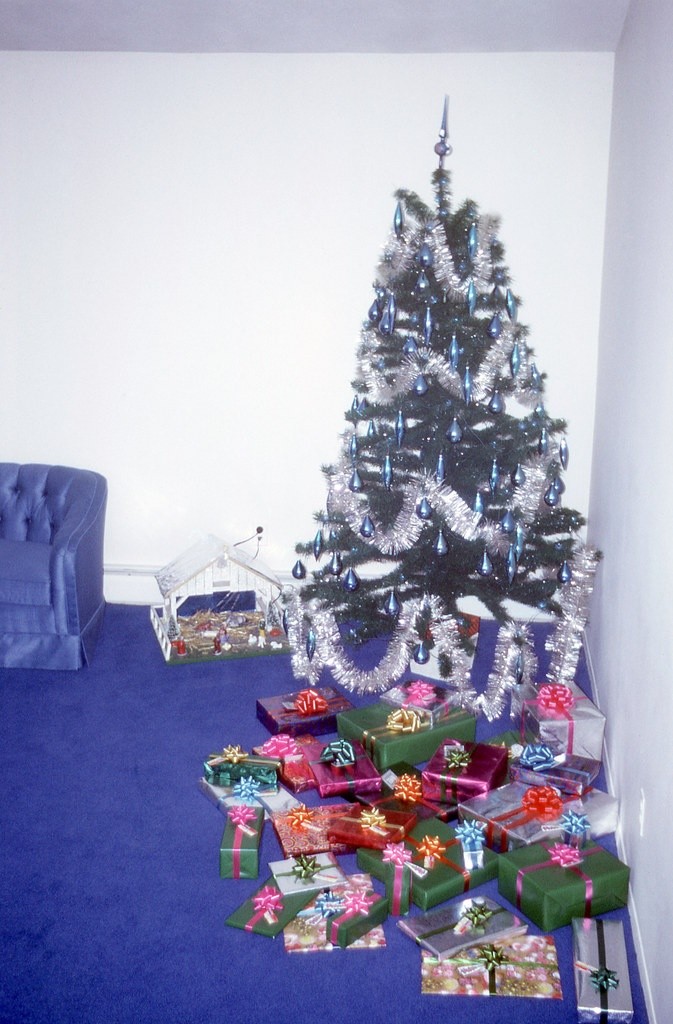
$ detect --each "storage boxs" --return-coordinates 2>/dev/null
[197,679,635,1023]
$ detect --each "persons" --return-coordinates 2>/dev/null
[213,627,232,653]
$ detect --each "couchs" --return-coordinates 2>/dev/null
[0,463,109,666]
